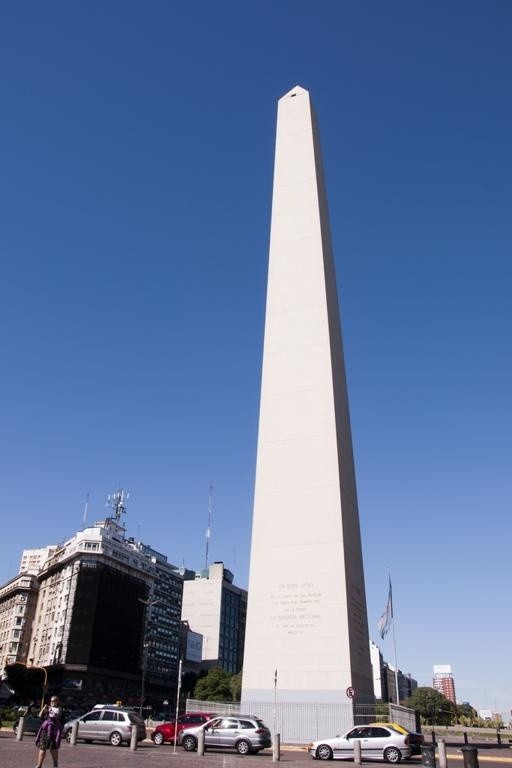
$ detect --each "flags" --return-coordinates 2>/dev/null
[377,575,393,641]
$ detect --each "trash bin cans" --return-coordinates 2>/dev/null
[460,747,480,768]
[420,744,436,768]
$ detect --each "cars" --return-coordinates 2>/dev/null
[13,704,146,746]
[151,712,271,755]
[308,722,424,763]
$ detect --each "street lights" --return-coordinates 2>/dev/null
[137,598,161,717]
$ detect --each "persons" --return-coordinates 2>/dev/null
[33,695,64,768]
